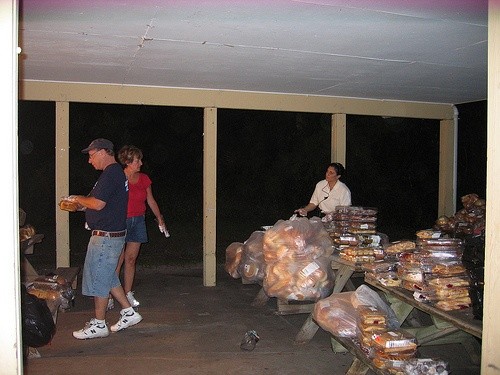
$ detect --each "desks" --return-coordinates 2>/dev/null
[254,253,484,375]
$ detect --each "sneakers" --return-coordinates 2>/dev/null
[105,298,114,311]
[110,309,144,332]
[126,291,140,308]
[73,321,109,340]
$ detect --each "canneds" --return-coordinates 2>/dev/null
[240,330,260,352]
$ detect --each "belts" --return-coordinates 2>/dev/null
[92,230,126,238]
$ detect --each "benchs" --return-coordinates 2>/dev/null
[313,319,389,375]
[48,267,80,324]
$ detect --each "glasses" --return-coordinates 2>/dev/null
[89,149,100,158]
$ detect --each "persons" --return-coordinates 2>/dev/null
[72,138,142,339]
[294,162,351,218]
[106,146,169,310]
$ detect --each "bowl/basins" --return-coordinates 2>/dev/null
[320,206,472,375]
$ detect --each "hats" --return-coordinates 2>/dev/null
[82,138,113,153]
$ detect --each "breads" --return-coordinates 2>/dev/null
[60,201,78,211]
[27,275,66,299]
[313,292,417,369]
[225,219,334,301]
[436,194,486,235]
[324,206,472,311]
[20,226,35,242]
